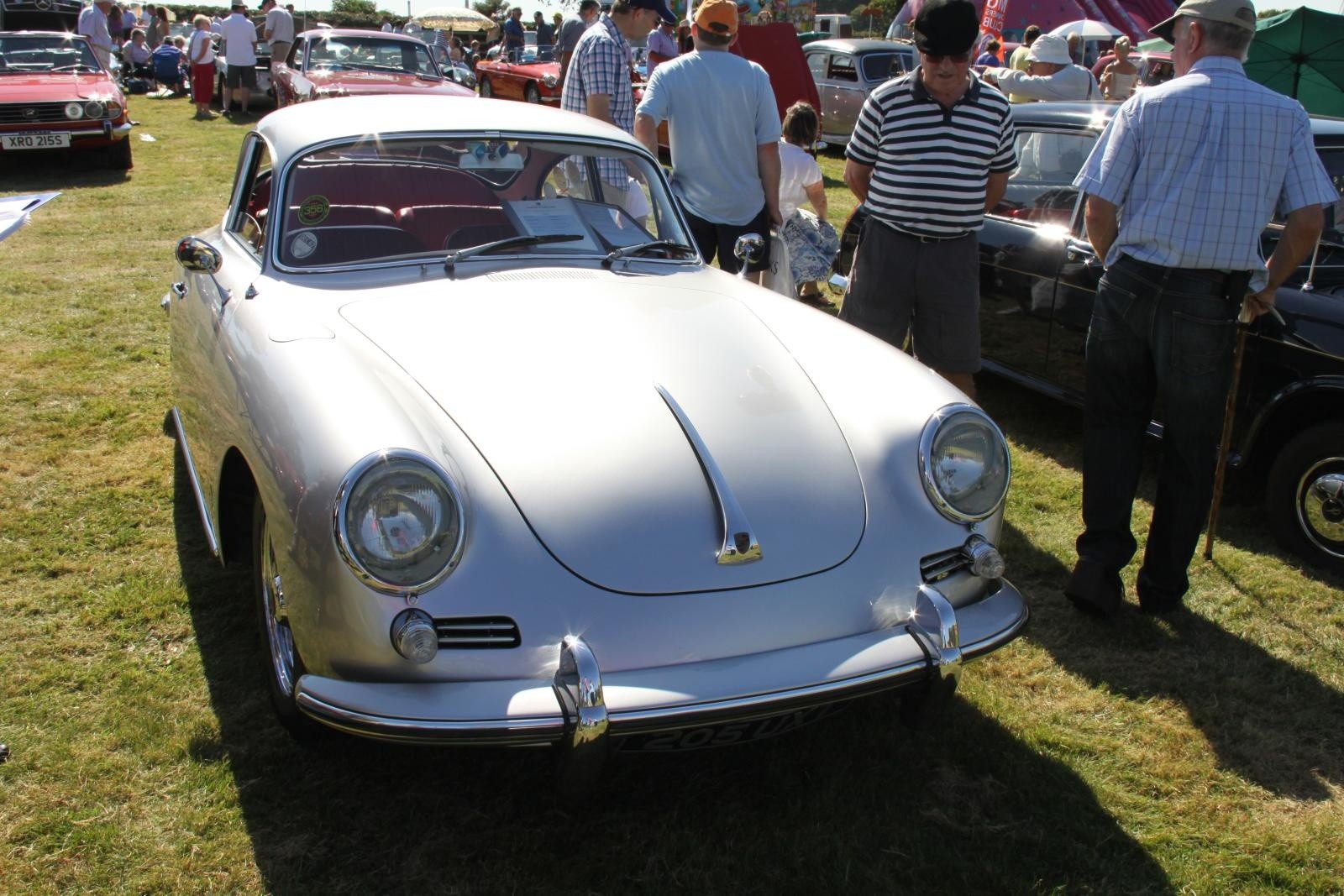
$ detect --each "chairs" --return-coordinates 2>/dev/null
[45,52,80,71]
[396,204,519,253]
[257,206,423,264]
[152,52,190,99]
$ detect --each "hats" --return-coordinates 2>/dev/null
[693,0,740,37]
[913,1,978,54]
[1024,34,1074,64]
[625,0,678,25]
[232,0,248,8]
[1147,1,1256,46]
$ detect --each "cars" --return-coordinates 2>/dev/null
[0,31,131,171]
[630,24,1344,565]
[138,18,563,111]
[158,94,1029,761]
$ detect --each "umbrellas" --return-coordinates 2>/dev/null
[1137,5,1344,119]
[413,7,496,37]
[1051,17,1124,68]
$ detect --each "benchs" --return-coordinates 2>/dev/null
[250,163,502,218]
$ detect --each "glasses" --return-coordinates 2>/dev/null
[924,51,976,63]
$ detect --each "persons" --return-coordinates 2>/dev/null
[79,0,223,125]
[646,18,695,81]
[257,0,294,107]
[376,7,525,64]
[561,0,678,207]
[777,101,836,306]
[286,4,294,13]
[634,0,783,284]
[221,0,258,115]
[974,25,1138,181]
[838,1,1018,397]
[1062,0,1338,616]
[533,0,639,88]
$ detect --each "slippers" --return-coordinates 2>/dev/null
[800,293,835,307]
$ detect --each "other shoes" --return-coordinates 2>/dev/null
[197,112,213,118]
[1140,595,1176,612]
[1065,563,1121,615]
[223,111,228,116]
[242,111,253,115]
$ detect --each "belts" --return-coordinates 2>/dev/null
[877,220,968,244]
